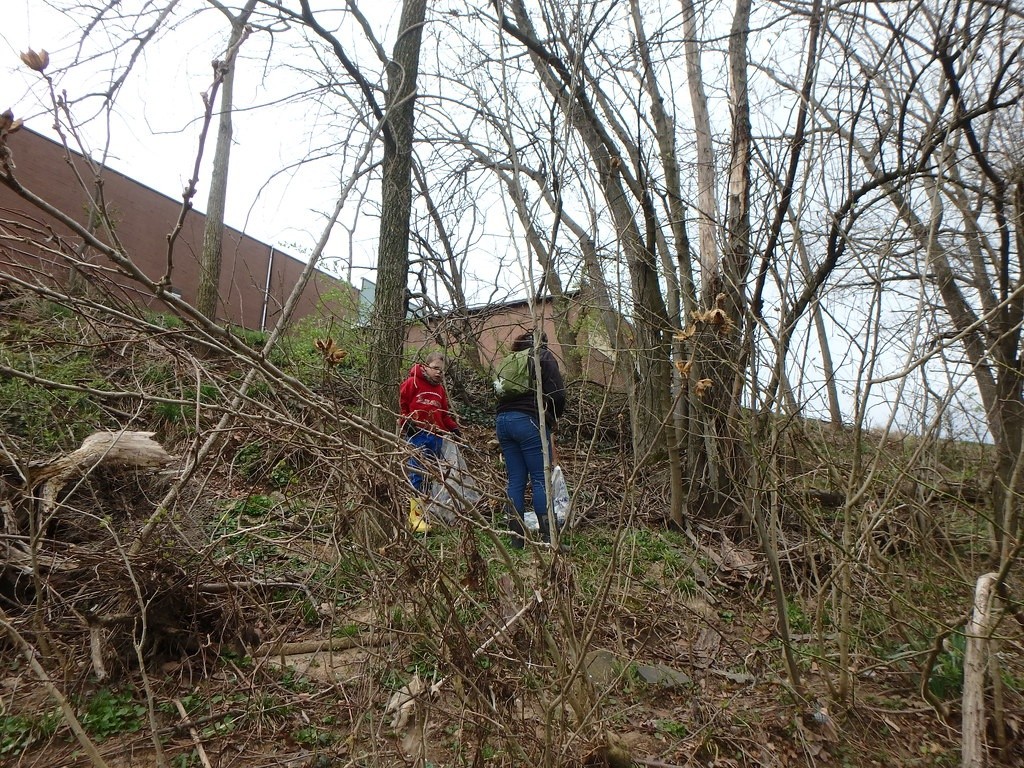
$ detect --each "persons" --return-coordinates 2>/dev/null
[400,352,460,532]
[496,329,572,554]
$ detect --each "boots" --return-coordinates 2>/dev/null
[409,497,433,532]
[508,513,525,550]
[538,511,569,551]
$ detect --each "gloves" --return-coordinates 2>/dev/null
[404,422,417,438]
[452,429,462,443]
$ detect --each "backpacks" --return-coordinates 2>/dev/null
[493,347,529,399]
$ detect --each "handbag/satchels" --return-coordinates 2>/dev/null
[551,464,569,527]
